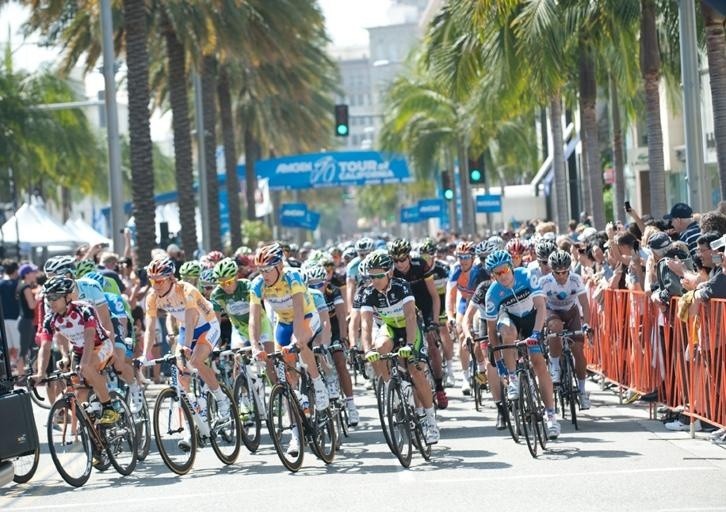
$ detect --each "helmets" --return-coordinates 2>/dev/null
[147,256,175,276]
[505,239,526,256]
[485,251,511,272]
[42,276,74,294]
[488,236,505,247]
[548,249,571,272]
[365,250,392,269]
[355,238,376,251]
[213,256,237,278]
[18,264,32,281]
[420,238,435,255]
[200,271,215,284]
[648,232,671,249]
[81,273,104,290]
[537,243,556,261]
[255,244,283,267]
[180,261,200,278]
[300,261,326,282]
[391,238,412,254]
[455,239,475,255]
[43,255,76,274]
[475,240,498,257]
[663,203,693,219]
[208,250,224,262]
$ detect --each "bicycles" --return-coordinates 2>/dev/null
[463,325,595,458]
[134,339,352,475]
[28,359,151,487]
[352,316,456,468]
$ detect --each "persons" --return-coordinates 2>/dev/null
[538,250,594,411]
[346,237,375,354]
[76,261,131,318]
[305,264,359,427]
[140,255,232,420]
[208,257,287,420]
[198,270,217,300]
[42,254,115,346]
[417,240,456,388]
[556,222,608,284]
[467,241,499,294]
[208,258,273,380]
[444,240,479,395]
[82,272,143,415]
[26,275,121,467]
[391,239,449,411]
[358,251,441,444]
[504,237,525,267]
[321,255,346,309]
[461,259,508,431]
[530,241,558,289]
[483,249,561,439]
[591,201,726,450]
[308,287,339,401]
[177,261,201,286]
[248,245,330,457]
[16,264,41,388]
[0,259,22,353]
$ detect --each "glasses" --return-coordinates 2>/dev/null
[149,277,170,285]
[309,282,323,289]
[694,248,711,253]
[554,270,567,275]
[368,270,391,280]
[494,265,510,276]
[46,294,65,301]
[460,255,470,260]
[258,267,275,273]
[392,255,408,263]
[218,279,235,288]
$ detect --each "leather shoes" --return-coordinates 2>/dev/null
[640,391,658,401]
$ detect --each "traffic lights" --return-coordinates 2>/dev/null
[466,143,486,185]
[467,146,485,184]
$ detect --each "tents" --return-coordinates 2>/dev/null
[0,203,78,264]
[35,213,114,256]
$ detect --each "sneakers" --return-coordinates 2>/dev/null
[578,390,591,411]
[324,376,341,400]
[462,378,470,393]
[287,422,300,456]
[98,402,121,425]
[437,391,448,410]
[545,412,560,439]
[665,416,702,432]
[623,393,639,405]
[545,359,561,387]
[216,395,230,421]
[425,417,440,448]
[91,458,100,466]
[311,379,329,412]
[242,415,255,429]
[506,375,519,400]
[178,429,192,454]
[347,407,359,426]
[274,394,284,416]
[497,401,507,429]
[128,377,143,415]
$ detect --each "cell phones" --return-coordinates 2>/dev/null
[625,201,631,212]
[632,239,639,251]
[710,254,722,264]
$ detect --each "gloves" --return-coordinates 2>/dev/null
[364,349,381,362]
[399,345,412,358]
[495,359,510,380]
[525,331,542,347]
